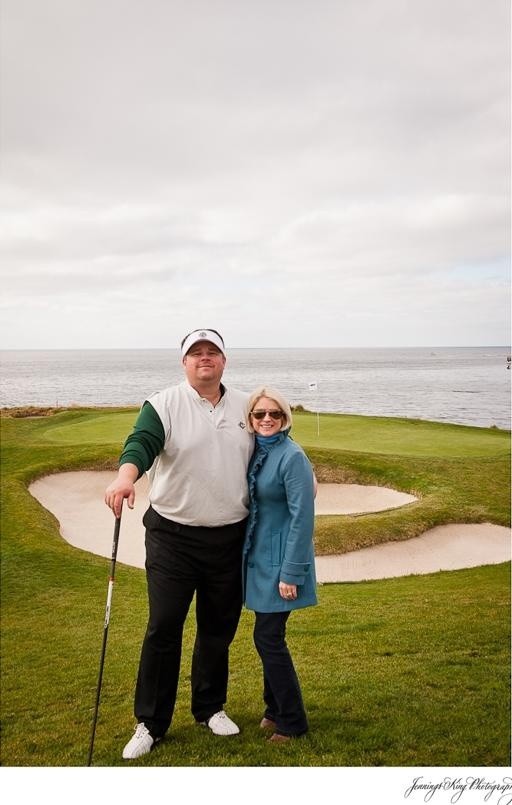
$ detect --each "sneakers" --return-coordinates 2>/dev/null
[122,722,162,759]
[266,733,291,744]
[259,717,278,730]
[195,709,241,736]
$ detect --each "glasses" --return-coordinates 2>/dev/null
[249,410,285,420]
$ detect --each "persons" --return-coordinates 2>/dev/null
[239,384,317,747]
[106,328,317,759]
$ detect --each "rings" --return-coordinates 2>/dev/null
[286,592,293,595]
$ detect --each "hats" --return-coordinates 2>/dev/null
[181,330,226,360]
